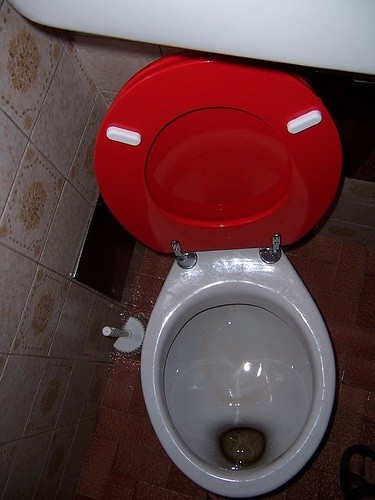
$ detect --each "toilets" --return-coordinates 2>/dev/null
[94,51,343,500]
